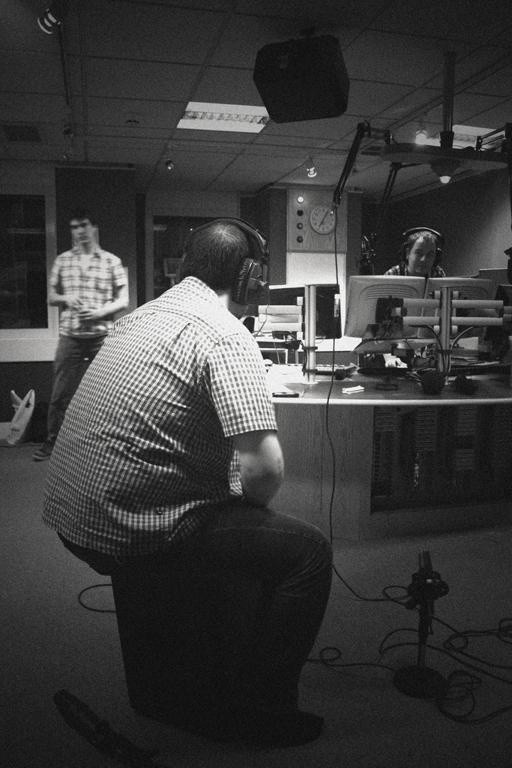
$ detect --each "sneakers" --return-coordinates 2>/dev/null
[29,448,51,461]
[246,707,327,751]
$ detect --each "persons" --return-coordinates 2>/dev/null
[380,225,448,279]
[32,207,128,462]
[38,216,333,749]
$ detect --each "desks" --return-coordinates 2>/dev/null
[269,365,511,536]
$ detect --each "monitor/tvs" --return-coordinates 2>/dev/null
[344,274,426,341]
[417,277,499,339]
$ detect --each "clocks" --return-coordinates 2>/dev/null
[310,203,338,236]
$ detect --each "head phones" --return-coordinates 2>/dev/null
[174,219,272,305]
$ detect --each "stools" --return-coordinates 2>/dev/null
[109,564,273,742]
